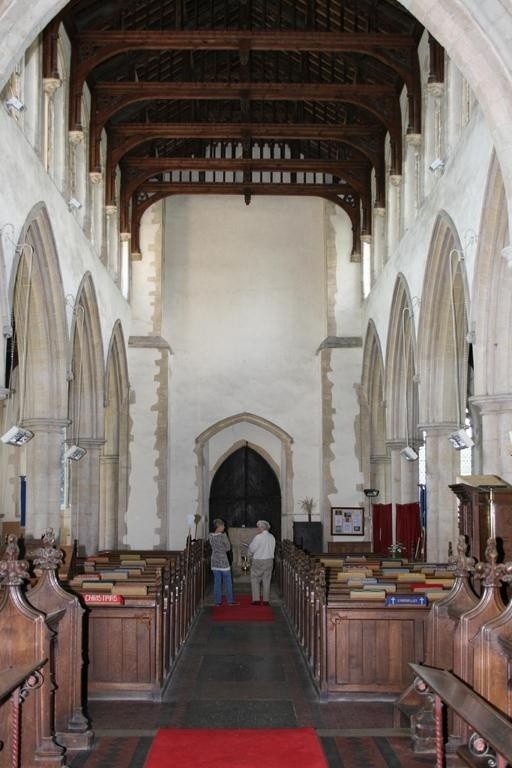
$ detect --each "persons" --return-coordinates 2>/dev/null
[246,519,276,607]
[207,518,241,607]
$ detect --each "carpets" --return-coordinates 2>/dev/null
[145,726,329,768]
[211,594,275,621]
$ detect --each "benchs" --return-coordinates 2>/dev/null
[275,539,457,703]
[23,526,213,703]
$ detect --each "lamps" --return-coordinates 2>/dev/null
[398,446,418,463]
[1,426,35,448]
[446,429,476,450]
[62,445,86,464]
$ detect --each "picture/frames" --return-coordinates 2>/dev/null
[331,507,365,536]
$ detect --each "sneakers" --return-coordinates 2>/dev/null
[227,602,240,607]
[263,601,269,606]
[216,600,225,608]
[248,601,261,606]
